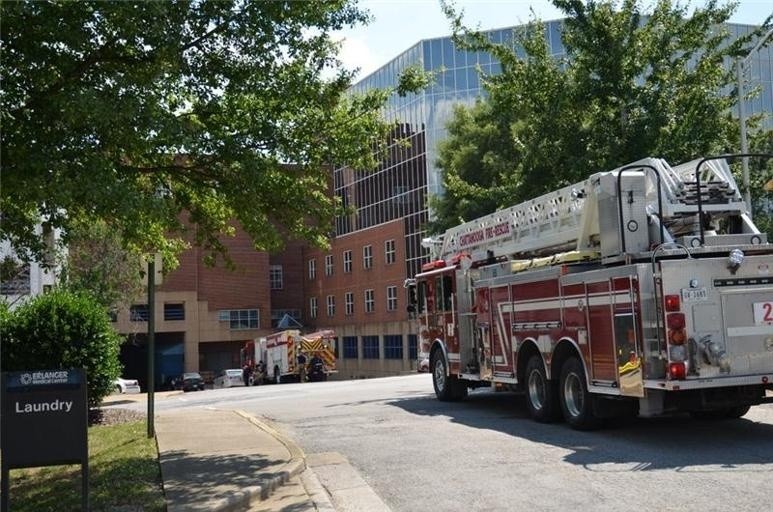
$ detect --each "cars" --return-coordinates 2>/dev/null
[103,375,142,396]
[199,370,216,384]
[172,372,204,391]
[210,367,246,389]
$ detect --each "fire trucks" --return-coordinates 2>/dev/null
[398,150,773,432]
[236,327,339,386]
